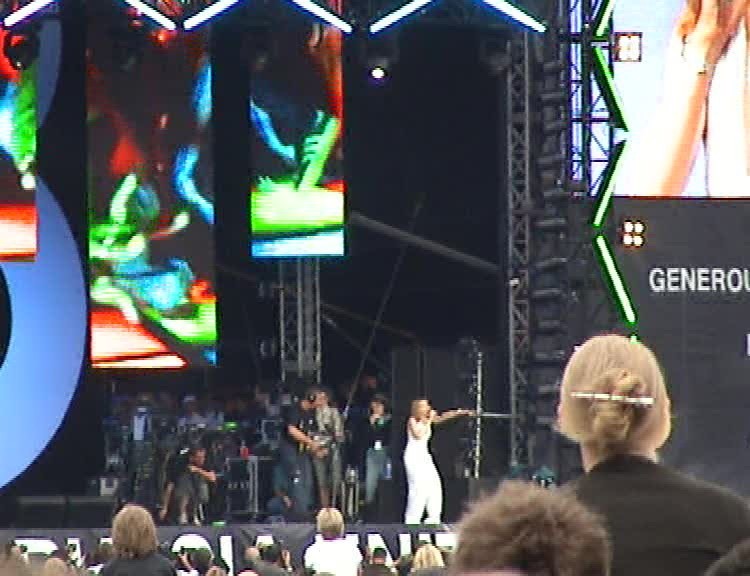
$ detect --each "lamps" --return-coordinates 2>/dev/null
[532,320,568,335]
[365,55,392,89]
[534,154,568,168]
[530,349,563,365]
[541,119,568,132]
[540,190,571,203]
[532,384,555,400]
[533,216,567,233]
[617,215,649,249]
[539,60,564,73]
[614,31,642,63]
[529,287,565,302]
[537,90,570,105]
[533,415,555,430]
[531,257,563,278]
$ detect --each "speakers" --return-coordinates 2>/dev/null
[15,496,67,529]
[63,496,115,527]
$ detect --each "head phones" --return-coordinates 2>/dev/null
[307,382,316,403]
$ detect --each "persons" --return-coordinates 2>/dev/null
[614,0,750,197]
[554,335,750,576]
[0,372,750,576]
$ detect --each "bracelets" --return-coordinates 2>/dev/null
[695,62,713,77]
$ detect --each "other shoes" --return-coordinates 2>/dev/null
[180,512,188,525]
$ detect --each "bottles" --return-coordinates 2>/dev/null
[240,440,247,458]
[385,456,392,479]
